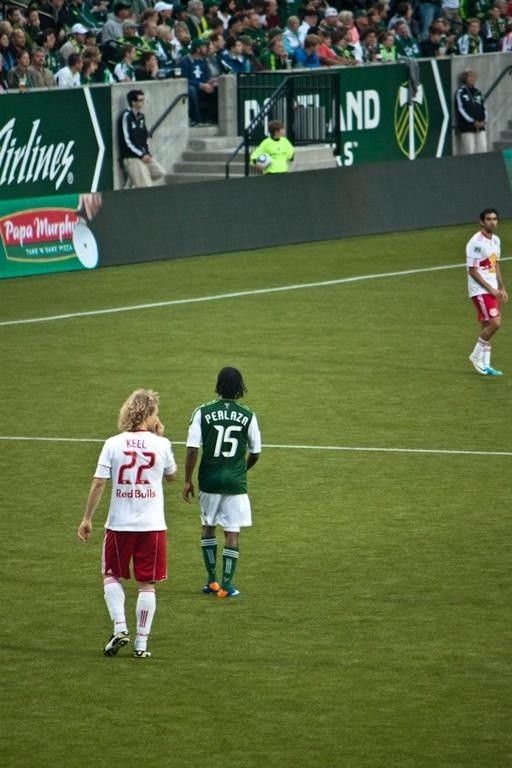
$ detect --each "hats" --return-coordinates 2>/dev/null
[114,2,132,11]
[154,2,174,12]
[71,23,89,35]
[325,7,338,18]
[354,9,373,18]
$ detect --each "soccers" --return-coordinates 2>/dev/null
[257,154,272,170]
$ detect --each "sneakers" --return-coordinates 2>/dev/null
[468,353,503,377]
[216,587,241,599]
[103,629,131,657]
[202,581,220,593]
[132,646,152,658]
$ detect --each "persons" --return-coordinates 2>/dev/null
[183,365,262,598]
[117,90,164,188]
[1,0,510,92]
[249,120,295,176]
[77,387,179,659]
[75,191,104,223]
[464,207,509,376]
[454,69,487,155]
